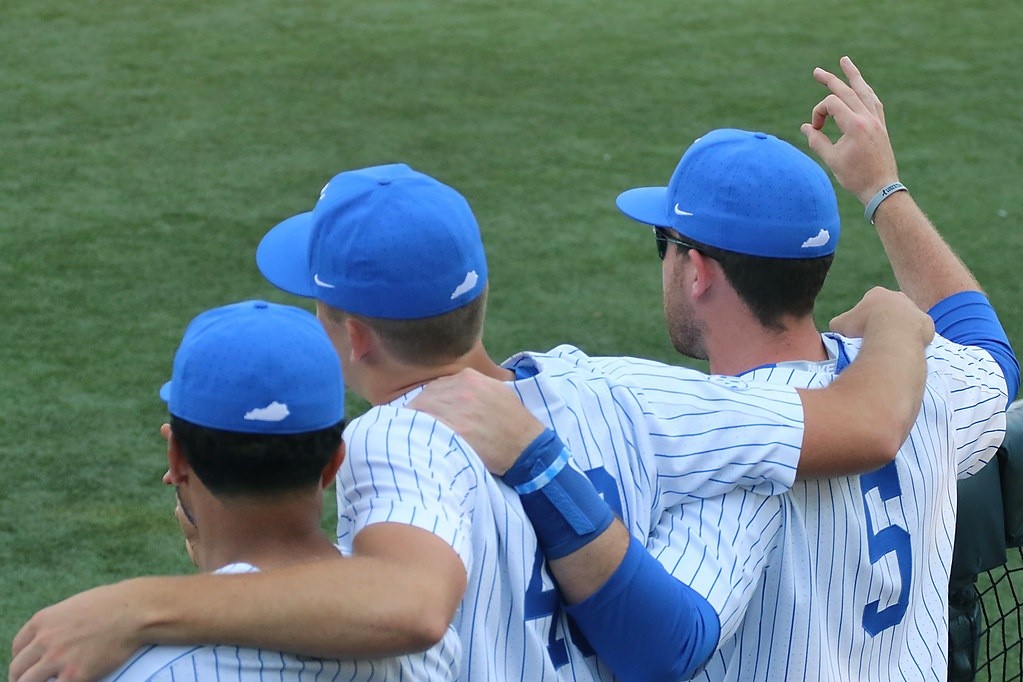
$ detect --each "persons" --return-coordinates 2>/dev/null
[44,301,464,682]
[8,164,935,682]
[403,57,1022,682]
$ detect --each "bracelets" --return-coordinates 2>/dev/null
[502,426,615,561]
[864,182,908,225]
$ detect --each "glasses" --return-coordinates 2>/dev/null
[652,225,722,262]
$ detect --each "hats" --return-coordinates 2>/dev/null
[159,299,345,434]
[616,128,841,259]
[255,163,488,321]
